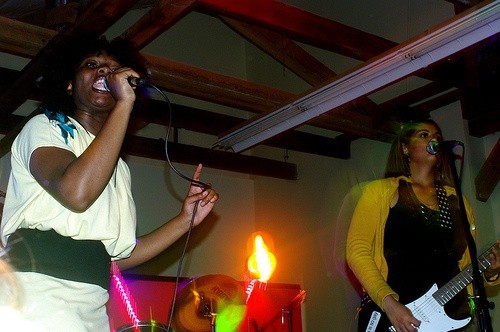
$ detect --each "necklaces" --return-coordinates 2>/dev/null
[413,185,438,205]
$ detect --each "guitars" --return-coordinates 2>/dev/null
[358,239,500,332]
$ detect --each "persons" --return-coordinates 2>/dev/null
[0,37,218,332]
[346,118,500,332]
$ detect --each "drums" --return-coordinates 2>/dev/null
[117,322,170,332]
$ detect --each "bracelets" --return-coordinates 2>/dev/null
[487,274,499,281]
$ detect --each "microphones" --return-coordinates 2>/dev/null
[427,138,463,155]
[103,73,149,92]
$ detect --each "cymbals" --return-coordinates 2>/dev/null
[172,274,245,332]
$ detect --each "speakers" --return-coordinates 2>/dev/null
[457,38,500,138]
